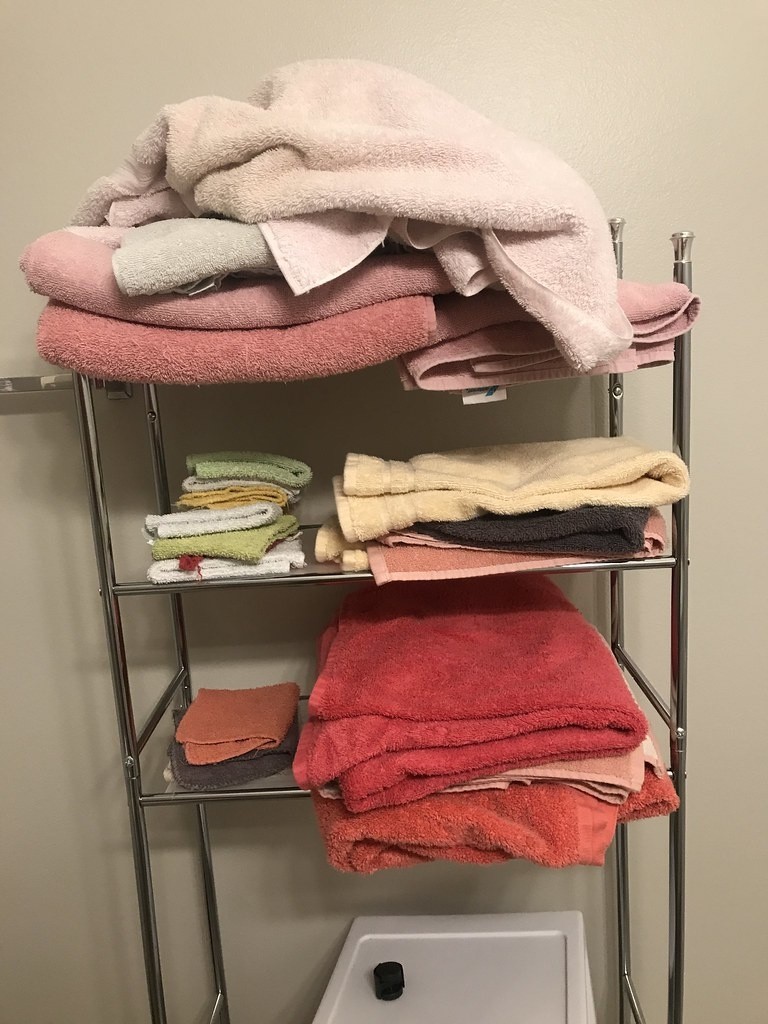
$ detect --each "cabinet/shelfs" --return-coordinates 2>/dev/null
[72,220,694,1024]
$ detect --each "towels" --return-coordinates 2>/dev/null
[174,681,301,766]
[313,434,690,586]
[292,573,650,814]
[311,621,666,806]
[141,502,307,586]
[166,705,300,791]
[310,722,680,876]
[392,278,703,393]
[171,449,313,510]
[16,53,630,386]
